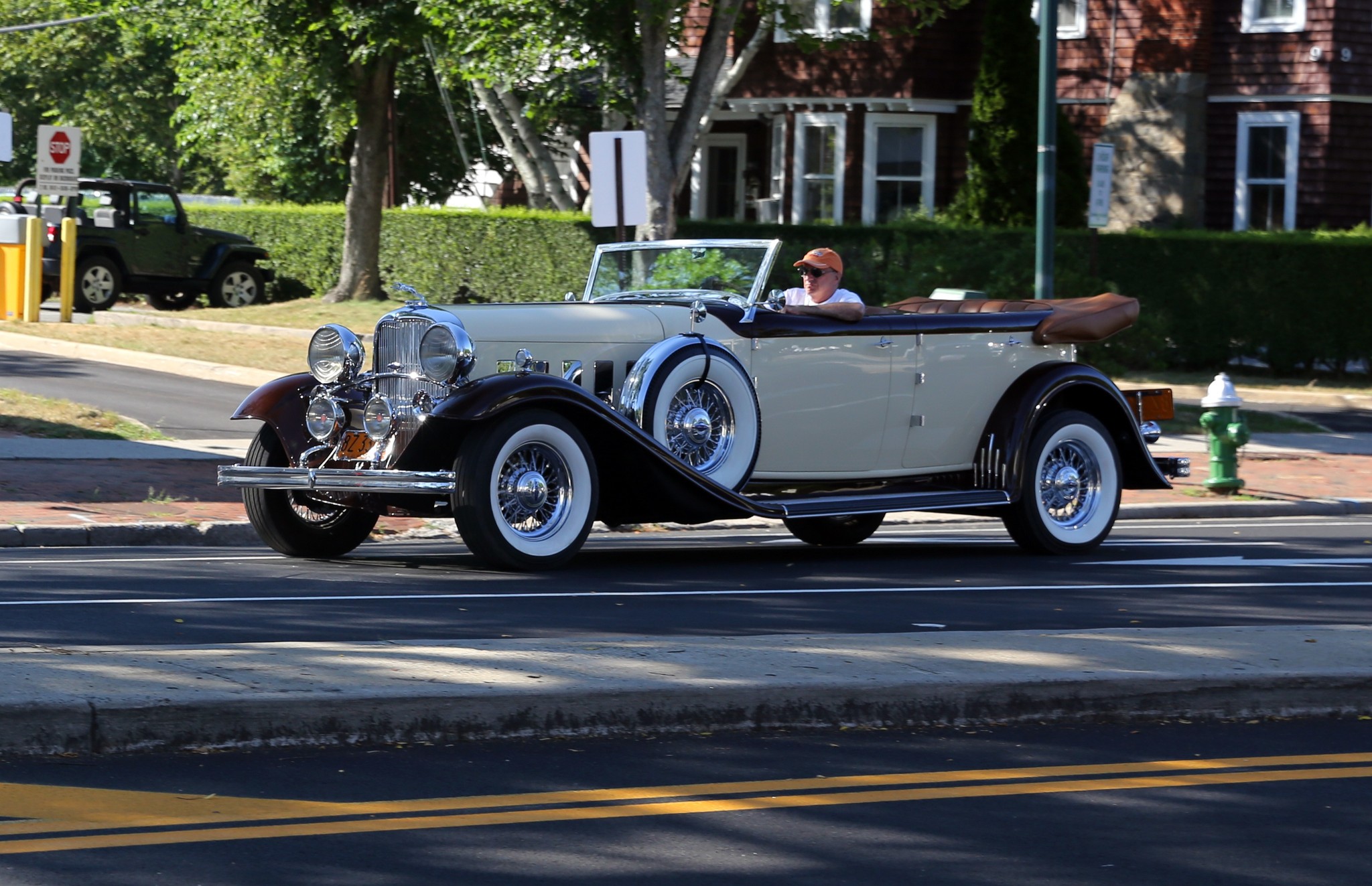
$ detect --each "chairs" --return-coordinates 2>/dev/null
[94,208,120,227]
[23,204,37,217]
[77,193,86,217]
[41,205,66,223]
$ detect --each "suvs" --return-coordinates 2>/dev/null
[0,177,276,314]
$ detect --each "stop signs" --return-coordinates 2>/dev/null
[49,130,71,164]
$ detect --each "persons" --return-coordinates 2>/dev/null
[763,248,865,322]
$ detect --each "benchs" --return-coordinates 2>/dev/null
[885,300,1052,313]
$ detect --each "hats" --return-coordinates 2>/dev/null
[793,248,843,275]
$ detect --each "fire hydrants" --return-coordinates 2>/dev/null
[1199,370,1251,495]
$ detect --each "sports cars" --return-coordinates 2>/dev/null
[214,237,1193,574]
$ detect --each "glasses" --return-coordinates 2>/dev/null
[797,266,838,279]
